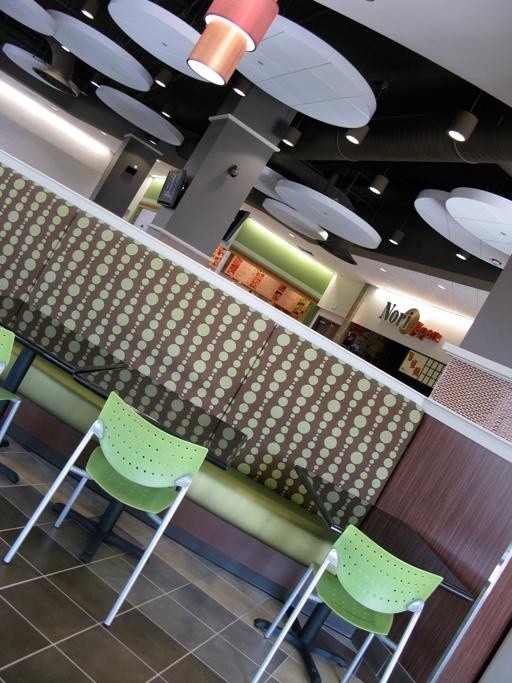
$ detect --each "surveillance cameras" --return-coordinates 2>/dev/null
[230,165,240,178]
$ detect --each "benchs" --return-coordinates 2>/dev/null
[1,149,424,639]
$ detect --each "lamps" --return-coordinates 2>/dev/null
[60,0,482,261]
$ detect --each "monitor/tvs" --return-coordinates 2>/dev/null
[157,169,187,208]
[223,210,251,241]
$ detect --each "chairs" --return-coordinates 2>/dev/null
[0,327,21,441]
[5,390,209,630]
[251,524,444,683]
